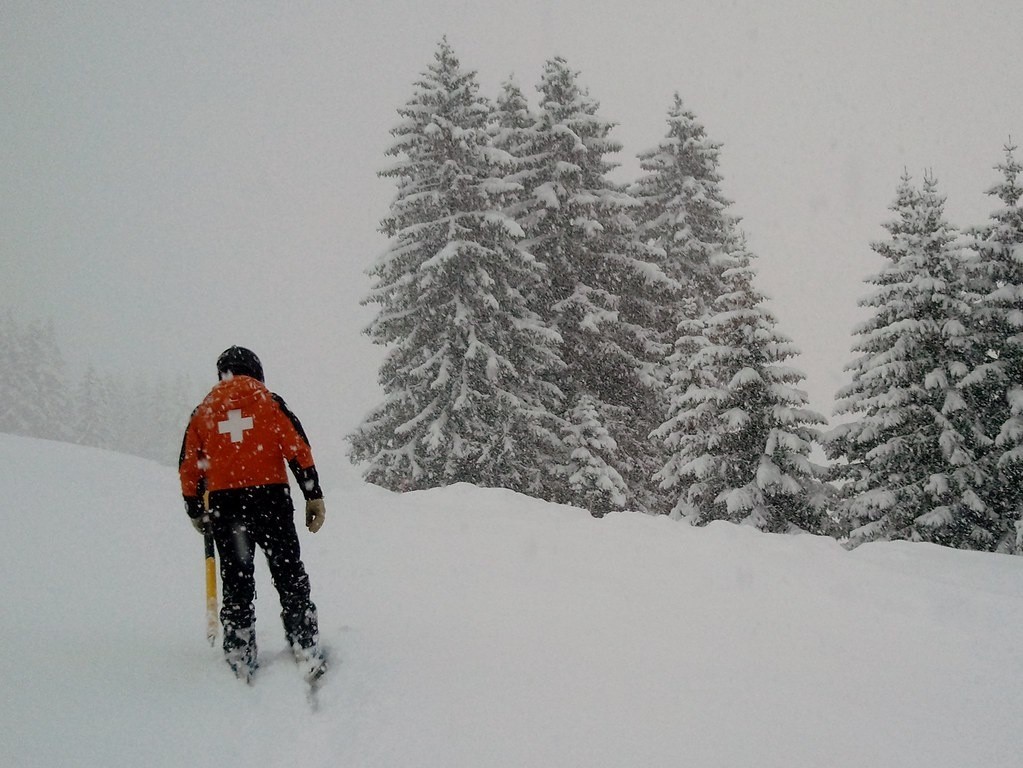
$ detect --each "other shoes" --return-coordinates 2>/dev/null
[224,652,258,686]
[290,638,324,680]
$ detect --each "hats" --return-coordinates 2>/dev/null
[216,346,264,384]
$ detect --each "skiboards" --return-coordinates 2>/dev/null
[230,608,327,686]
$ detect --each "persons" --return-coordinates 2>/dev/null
[179,346,325,673]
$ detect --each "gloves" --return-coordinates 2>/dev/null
[192,514,205,533]
[305,499,326,535]
